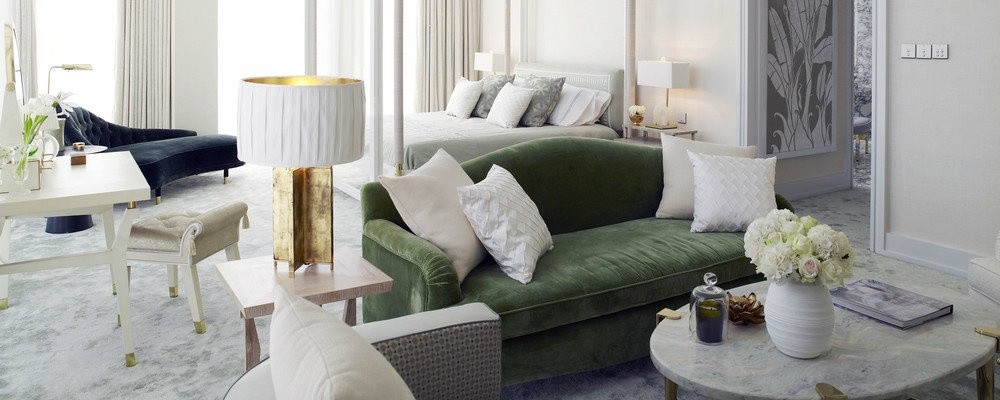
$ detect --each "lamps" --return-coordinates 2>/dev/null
[47,64,93,96]
[237,76,366,278]
[637,60,690,129]
[474,52,505,75]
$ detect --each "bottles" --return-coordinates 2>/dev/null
[689,272,732,346]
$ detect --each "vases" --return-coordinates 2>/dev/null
[764,276,836,359]
[9,147,32,197]
[629,116,644,126]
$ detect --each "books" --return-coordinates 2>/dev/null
[830,278,953,331]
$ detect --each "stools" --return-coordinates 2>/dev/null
[213,252,393,371]
[113,202,248,334]
[853,118,871,162]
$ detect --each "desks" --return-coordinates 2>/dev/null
[649,272,1000,400]
[0,150,151,367]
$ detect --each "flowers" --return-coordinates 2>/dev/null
[630,104,645,115]
[11,91,78,145]
[745,208,857,288]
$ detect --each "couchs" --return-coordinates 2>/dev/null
[64,106,247,209]
[220,136,796,400]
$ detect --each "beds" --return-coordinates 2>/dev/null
[383,63,624,172]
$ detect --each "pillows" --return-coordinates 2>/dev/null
[445,74,566,128]
[270,285,416,400]
[377,134,779,285]
